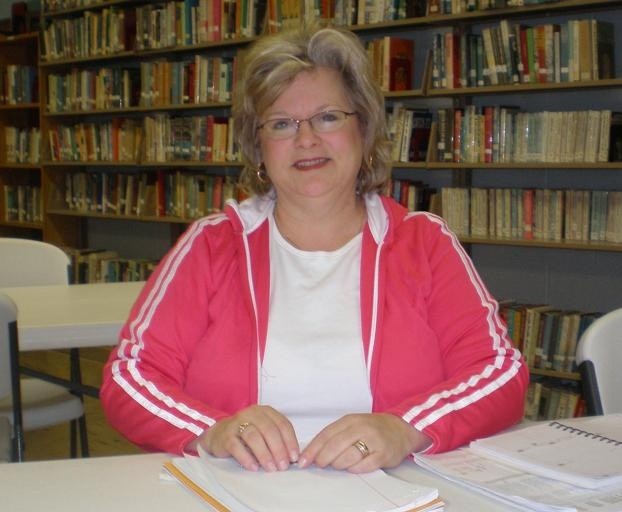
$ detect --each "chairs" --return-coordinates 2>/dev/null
[0,238,89,463]
[572,304,621,416]
[1,291,32,463]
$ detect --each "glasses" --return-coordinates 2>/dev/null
[257,110,358,139]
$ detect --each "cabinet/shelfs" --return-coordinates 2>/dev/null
[0,2,622,382]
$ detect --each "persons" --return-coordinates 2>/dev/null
[98,21,529,474]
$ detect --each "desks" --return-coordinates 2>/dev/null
[0,417,621,511]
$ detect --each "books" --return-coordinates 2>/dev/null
[42,2,622,244]
[470,421,622,489]
[495,295,602,418]
[62,246,160,284]
[1,2,40,224]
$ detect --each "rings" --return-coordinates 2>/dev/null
[238,422,249,439]
[352,439,370,458]
